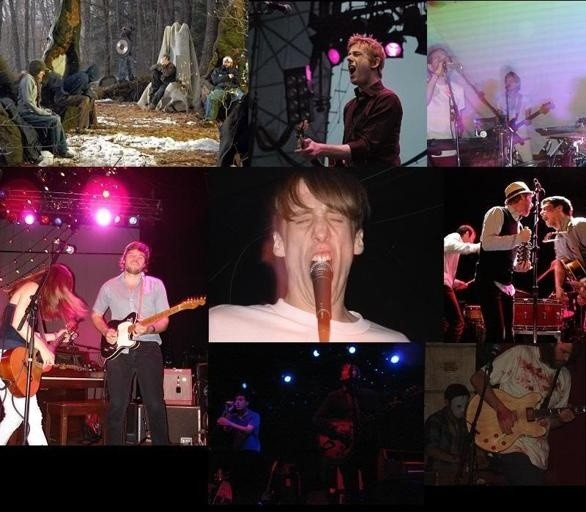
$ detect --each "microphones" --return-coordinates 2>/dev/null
[51,237,75,255]
[307,258,334,346]
[484,341,503,372]
[224,402,241,407]
[532,178,547,199]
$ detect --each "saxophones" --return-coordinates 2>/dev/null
[516,226,531,270]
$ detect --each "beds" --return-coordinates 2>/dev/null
[38,375,105,438]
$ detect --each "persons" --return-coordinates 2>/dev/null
[424,384,473,486]
[148,54,177,111]
[294,33,402,168]
[202,55,245,128]
[540,196,586,302]
[426,48,466,166]
[53,64,107,134]
[474,181,535,343]
[18,60,75,158]
[1,263,90,446]
[313,364,402,505]
[208,167,415,343]
[90,242,170,445]
[217,393,261,503]
[470,343,576,486]
[444,224,481,343]
[496,72,536,167]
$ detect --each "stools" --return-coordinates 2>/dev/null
[44,399,108,446]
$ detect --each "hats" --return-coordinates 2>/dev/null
[504,181,535,204]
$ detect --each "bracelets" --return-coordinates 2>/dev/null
[151,325,156,334]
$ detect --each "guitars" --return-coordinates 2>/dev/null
[318,385,419,460]
[100,296,205,361]
[0,317,78,398]
[464,388,585,455]
[558,255,585,302]
[472,100,555,150]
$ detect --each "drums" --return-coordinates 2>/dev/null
[511,298,560,335]
[465,305,485,341]
